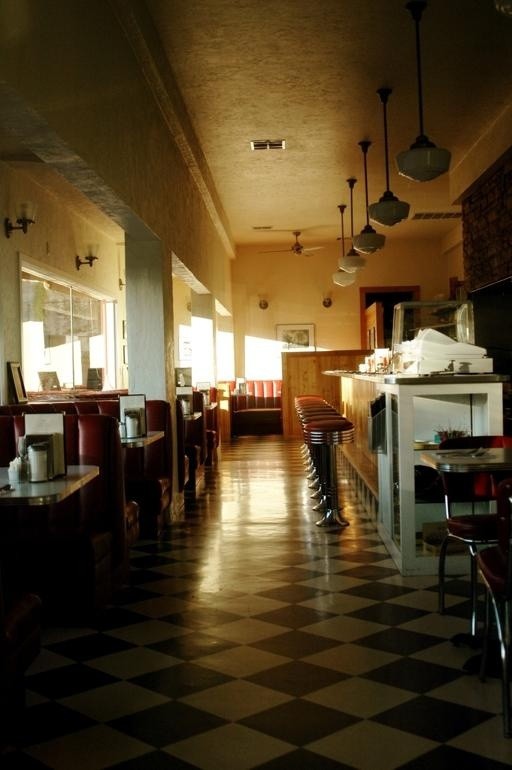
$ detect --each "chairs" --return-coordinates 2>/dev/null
[420,437,512,738]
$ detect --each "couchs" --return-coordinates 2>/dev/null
[218,380,283,441]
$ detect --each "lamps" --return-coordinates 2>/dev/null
[258,290,269,309]
[321,288,332,308]
[76,242,100,270]
[332,0,451,288]
[5,201,36,239]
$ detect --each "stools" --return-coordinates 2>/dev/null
[294,395,355,528]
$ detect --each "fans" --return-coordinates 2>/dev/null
[258,232,328,257]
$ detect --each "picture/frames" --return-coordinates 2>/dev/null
[276,324,315,353]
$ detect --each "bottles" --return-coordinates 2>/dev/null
[117,421,126,439]
